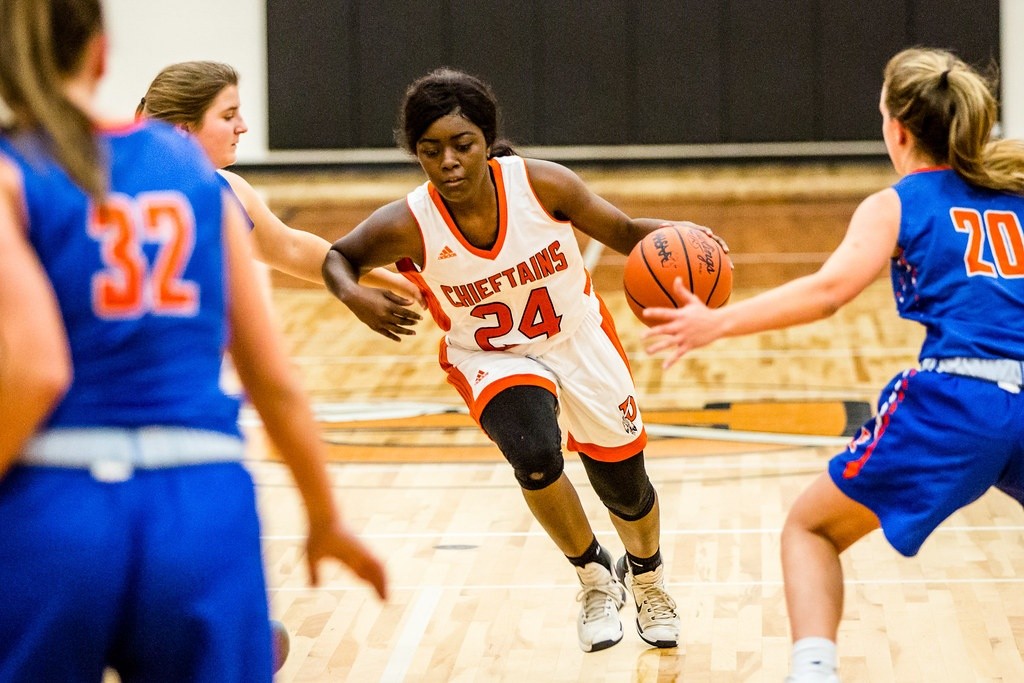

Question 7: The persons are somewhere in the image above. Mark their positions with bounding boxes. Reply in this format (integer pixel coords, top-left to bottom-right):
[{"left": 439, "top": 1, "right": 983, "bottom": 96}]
[
  {"left": 322, "top": 69, "right": 733, "bottom": 655},
  {"left": 0, "top": 0, "right": 388, "bottom": 683},
  {"left": 637, "top": 48, "right": 1024, "bottom": 681},
  {"left": 128, "top": 64, "right": 434, "bottom": 308}
]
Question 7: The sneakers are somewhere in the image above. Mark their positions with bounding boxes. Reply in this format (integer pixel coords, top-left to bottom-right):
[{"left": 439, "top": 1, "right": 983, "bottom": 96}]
[
  {"left": 575, "top": 562, "right": 626, "bottom": 654},
  {"left": 616, "top": 552, "right": 682, "bottom": 647}
]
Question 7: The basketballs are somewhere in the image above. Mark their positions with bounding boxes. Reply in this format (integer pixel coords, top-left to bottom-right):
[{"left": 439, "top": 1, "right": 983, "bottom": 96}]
[{"left": 620, "top": 224, "right": 733, "bottom": 336}]
